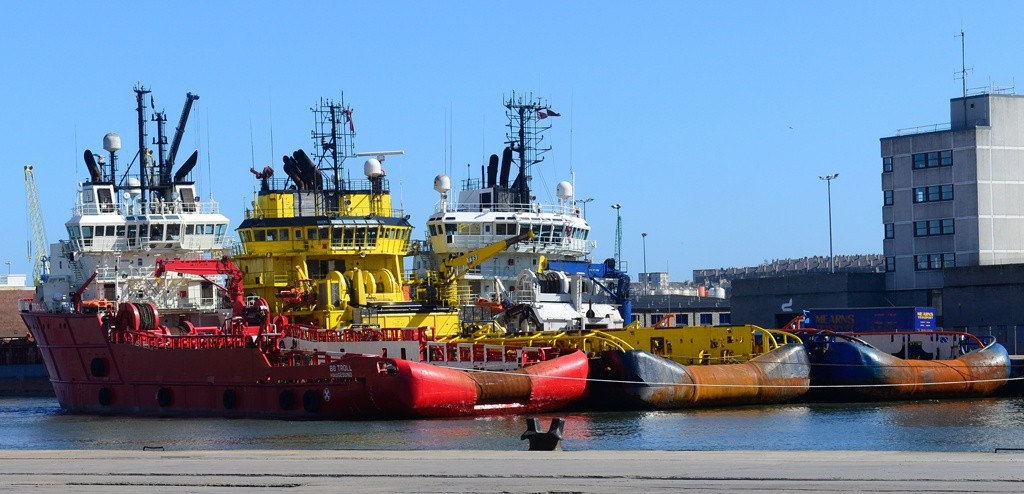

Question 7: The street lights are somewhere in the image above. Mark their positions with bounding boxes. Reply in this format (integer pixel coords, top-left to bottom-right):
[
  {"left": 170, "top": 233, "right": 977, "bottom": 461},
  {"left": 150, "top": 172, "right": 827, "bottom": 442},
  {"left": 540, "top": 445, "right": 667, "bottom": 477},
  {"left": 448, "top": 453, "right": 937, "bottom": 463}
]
[
  {"left": 818, "top": 172, "right": 842, "bottom": 275},
  {"left": 576, "top": 198, "right": 596, "bottom": 261},
  {"left": 642, "top": 233, "right": 648, "bottom": 295},
  {"left": 610, "top": 203, "right": 623, "bottom": 271}
]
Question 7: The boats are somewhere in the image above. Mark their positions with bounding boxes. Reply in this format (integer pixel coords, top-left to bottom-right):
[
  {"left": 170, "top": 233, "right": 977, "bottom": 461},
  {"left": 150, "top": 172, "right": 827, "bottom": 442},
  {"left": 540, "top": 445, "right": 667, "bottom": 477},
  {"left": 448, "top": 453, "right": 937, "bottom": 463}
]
[{"left": 17, "top": 76, "right": 1015, "bottom": 424}]
